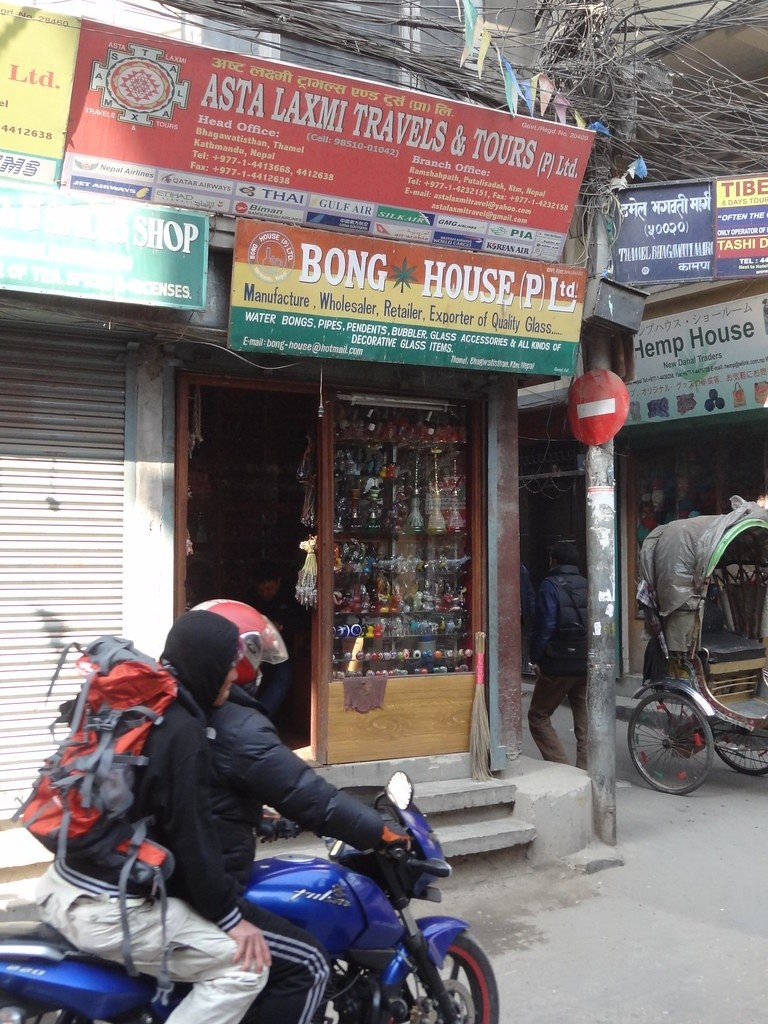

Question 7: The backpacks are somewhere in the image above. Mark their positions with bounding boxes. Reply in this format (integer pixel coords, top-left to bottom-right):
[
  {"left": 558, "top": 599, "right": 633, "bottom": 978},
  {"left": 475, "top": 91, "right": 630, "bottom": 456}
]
[{"left": 20, "top": 636, "right": 205, "bottom": 884}]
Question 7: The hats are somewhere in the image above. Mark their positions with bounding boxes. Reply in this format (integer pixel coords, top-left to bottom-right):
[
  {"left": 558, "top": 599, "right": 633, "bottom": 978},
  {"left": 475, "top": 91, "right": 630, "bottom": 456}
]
[{"left": 547, "top": 540, "right": 580, "bottom": 563}]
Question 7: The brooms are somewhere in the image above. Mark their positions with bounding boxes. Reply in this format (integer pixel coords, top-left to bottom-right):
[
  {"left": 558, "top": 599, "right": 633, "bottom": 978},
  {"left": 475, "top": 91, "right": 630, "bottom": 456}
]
[{"left": 470, "top": 632, "right": 494, "bottom": 782}]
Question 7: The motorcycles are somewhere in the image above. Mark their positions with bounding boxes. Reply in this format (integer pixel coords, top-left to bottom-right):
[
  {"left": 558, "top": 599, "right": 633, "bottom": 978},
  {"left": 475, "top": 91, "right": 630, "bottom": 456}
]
[{"left": 1, "top": 771, "right": 501, "bottom": 1023}]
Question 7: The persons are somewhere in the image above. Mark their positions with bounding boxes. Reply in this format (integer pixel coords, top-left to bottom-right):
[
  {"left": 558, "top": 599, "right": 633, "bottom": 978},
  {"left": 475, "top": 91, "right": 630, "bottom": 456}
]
[
  {"left": 39, "top": 598, "right": 413, "bottom": 1024},
  {"left": 518, "top": 539, "right": 588, "bottom": 771}
]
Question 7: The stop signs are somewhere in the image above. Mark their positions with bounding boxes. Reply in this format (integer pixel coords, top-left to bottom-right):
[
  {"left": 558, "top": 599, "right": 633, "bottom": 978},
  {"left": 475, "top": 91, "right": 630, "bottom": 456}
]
[{"left": 568, "top": 370, "right": 632, "bottom": 448}]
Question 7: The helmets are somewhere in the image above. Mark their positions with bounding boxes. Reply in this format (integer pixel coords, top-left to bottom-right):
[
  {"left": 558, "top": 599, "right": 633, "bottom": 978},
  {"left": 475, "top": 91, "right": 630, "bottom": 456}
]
[{"left": 189, "top": 599, "right": 290, "bottom": 697}]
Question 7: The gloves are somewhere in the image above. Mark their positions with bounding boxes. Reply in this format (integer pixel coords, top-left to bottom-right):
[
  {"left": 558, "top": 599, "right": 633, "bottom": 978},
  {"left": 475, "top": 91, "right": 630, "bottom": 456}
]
[
  {"left": 375, "top": 820, "right": 412, "bottom": 858},
  {"left": 262, "top": 804, "right": 283, "bottom": 840}
]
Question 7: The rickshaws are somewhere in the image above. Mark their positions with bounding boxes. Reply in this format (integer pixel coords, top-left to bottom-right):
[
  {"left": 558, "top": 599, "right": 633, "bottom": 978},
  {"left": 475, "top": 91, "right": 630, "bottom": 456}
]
[{"left": 624, "top": 502, "right": 767, "bottom": 795}]
